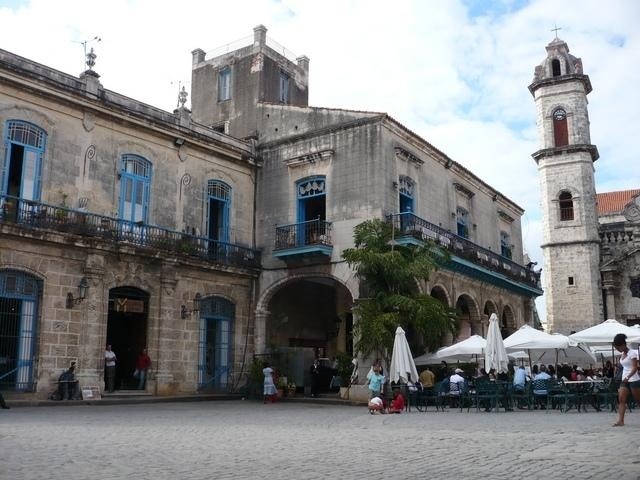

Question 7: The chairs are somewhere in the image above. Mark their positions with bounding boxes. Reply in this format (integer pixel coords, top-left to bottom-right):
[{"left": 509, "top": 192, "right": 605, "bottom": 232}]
[{"left": 382, "top": 371, "right": 640, "bottom": 417}]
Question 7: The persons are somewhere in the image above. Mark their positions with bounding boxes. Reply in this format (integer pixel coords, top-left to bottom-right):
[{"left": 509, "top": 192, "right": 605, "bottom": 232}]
[
  {"left": 262, "top": 362, "right": 279, "bottom": 404},
  {"left": 310, "top": 358, "right": 322, "bottom": 397},
  {"left": 365, "top": 333, "right": 640, "bottom": 426},
  {"left": 58, "top": 366, "right": 79, "bottom": 401},
  {"left": 133, "top": 348, "right": 151, "bottom": 390},
  {"left": 105, "top": 344, "right": 118, "bottom": 393}
]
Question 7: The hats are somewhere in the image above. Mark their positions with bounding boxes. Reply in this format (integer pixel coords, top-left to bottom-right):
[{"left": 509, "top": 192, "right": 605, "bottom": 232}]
[{"left": 455, "top": 368, "right": 464, "bottom": 374}]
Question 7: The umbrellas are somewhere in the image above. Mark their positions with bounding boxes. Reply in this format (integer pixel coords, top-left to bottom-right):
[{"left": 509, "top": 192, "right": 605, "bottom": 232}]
[{"left": 389, "top": 313, "right": 640, "bottom": 411}]
